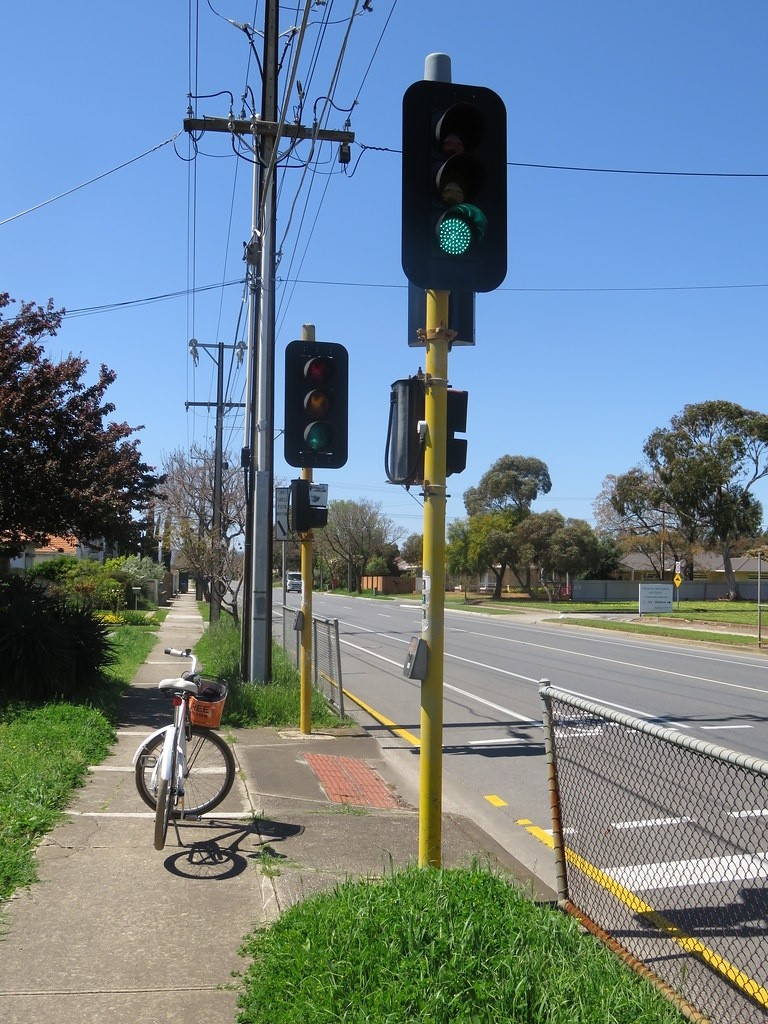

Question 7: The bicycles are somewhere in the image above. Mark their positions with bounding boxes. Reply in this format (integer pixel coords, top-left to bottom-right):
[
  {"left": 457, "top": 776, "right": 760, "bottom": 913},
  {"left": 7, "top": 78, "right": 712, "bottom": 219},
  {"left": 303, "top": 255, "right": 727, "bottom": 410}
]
[{"left": 131, "top": 646, "right": 237, "bottom": 849}]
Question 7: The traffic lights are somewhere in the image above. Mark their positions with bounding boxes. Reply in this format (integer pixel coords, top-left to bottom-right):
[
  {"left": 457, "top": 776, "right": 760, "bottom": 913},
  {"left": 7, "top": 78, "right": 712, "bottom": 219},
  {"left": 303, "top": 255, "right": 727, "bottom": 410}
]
[
  {"left": 284, "top": 340, "right": 348, "bottom": 469},
  {"left": 384, "top": 376, "right": 467, "bottom": 484},
  {"left": 289, "top": 479, "right": 332, "bottom": 535},
  {"left": 401, "top": 79, "right": 509, "bottom": 291}
]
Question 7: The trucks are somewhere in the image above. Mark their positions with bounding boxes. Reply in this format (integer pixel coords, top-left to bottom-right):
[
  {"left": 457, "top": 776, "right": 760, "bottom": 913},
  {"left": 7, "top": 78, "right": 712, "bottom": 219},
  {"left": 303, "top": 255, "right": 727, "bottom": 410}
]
[{"left": 287, "top": 571, "right": 301, "bottom": 594}]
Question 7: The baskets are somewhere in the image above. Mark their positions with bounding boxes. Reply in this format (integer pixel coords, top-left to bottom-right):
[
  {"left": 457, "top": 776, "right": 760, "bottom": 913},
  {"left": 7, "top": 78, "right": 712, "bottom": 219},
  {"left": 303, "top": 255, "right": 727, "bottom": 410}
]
[{"left": 189, "top": 679, "right": 227, "bottom": 728}]
